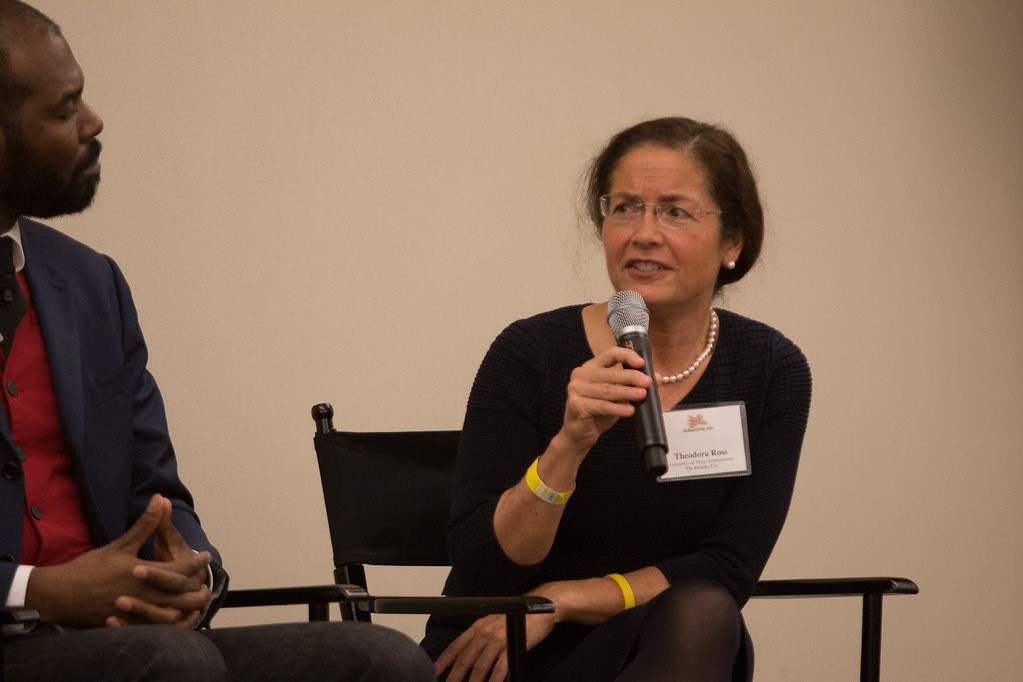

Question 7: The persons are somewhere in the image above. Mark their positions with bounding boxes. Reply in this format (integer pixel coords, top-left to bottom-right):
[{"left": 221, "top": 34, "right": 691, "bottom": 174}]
[
  {"left": 420, "top": 117, "right": 813, "bottom": 682},
  {"left": 0, "top": 0, "right": 448, "bottom": 682}
]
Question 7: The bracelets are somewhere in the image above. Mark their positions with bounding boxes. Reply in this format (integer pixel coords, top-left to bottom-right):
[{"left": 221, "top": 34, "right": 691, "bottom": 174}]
[
  {"left": 525, "top": 455, "right": 577, "bottom": 505},
  {"left": 604, "top": 572, "right": 636, "bottom": 611}
]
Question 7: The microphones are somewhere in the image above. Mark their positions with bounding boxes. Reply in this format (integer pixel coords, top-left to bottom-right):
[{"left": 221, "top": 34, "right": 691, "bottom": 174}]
[{"left": 607, "top": 289, "right": 671, "bottom": 477}]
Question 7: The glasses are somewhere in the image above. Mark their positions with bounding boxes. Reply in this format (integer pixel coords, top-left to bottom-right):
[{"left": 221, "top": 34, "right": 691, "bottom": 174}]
[{"left": 599, "top": 193, "right": 725, "bottom": 229}]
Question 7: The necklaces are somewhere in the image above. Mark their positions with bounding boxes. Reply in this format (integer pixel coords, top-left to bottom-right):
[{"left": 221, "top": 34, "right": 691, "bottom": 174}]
[{"left": 661, "top": 304, "right": 718, "bottom": 384}]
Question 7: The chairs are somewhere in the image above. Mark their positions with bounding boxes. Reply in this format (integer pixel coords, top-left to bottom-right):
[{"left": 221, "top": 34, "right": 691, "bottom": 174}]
[{"left": 309, "top": 399, "right": 920, "bottom": 682}]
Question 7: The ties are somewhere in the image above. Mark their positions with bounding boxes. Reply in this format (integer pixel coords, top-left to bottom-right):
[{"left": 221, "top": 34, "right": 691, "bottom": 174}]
[{"left": 0, "top": 236, "right": 26, "bottom": 360}]
